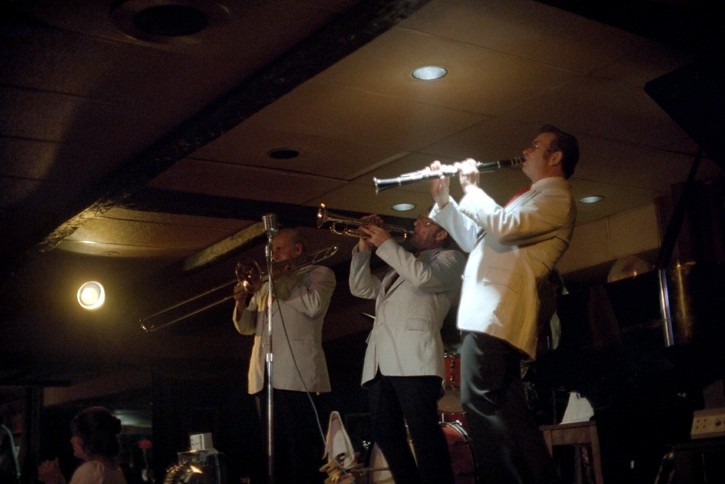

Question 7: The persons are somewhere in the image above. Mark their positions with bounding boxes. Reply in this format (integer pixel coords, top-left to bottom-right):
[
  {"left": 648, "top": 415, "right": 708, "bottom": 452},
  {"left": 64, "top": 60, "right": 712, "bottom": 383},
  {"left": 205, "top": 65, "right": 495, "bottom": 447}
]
[
  {"left": 38, "top": 407, "right": 127, "bottom": 484},
  {"left": 349, "top": 203, "right": 459, "bottom": 484},
  {"left": 233, "top": 228, "right": 336, "bottom": 484},
  {"left": 426, "top": 125, "right": 579, "bottom": 484}
]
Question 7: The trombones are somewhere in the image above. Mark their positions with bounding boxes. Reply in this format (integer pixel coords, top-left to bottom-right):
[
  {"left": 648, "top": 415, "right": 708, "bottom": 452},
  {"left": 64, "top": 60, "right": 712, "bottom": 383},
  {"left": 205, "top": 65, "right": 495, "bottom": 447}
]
[{"left": 135, "top": 247, "right": 338, "bottom": 334}]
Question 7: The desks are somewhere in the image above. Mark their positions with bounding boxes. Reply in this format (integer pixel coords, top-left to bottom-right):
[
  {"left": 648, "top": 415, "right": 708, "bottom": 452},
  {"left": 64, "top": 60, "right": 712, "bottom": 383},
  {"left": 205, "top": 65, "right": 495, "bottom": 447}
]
[{"left": 539, "top": 421, "right": 603, "bottom": 484}]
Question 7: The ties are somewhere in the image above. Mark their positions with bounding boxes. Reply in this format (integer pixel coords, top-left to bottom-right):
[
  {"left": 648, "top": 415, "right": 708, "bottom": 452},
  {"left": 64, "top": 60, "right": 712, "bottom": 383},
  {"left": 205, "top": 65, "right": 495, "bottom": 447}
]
[{"left": 506, "top": 187, "right": 531, "bottom": 207}]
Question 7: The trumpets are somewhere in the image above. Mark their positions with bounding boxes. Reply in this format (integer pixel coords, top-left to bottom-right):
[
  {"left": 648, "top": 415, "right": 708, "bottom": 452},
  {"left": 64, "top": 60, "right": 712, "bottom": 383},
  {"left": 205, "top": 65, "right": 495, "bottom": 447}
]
[
  {"left": 373, "top": 156, "right": 523, "bottom": 193},
  {"left": 315, "top": 203, "right": 416, "bottom": 241}
]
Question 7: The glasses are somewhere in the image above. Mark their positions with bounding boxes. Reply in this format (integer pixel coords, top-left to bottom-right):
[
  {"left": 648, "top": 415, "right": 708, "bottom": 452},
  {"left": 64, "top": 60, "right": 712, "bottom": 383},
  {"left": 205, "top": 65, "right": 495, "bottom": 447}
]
[{"left": 418, "top": 214, "right": 444, "bottom": 230}]
